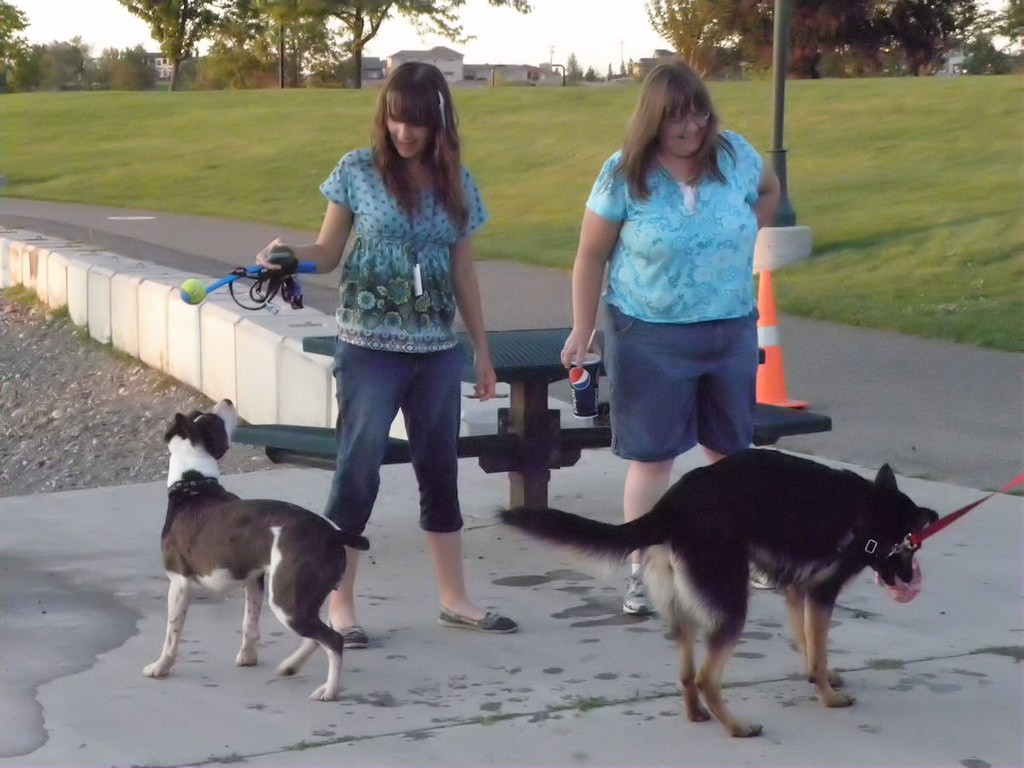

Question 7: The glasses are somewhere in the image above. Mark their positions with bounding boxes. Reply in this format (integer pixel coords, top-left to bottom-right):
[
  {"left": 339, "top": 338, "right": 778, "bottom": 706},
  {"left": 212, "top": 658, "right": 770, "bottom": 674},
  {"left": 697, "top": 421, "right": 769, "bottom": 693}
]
[{"left": 666, "top": 109, "right": 710, "bottom": 128}]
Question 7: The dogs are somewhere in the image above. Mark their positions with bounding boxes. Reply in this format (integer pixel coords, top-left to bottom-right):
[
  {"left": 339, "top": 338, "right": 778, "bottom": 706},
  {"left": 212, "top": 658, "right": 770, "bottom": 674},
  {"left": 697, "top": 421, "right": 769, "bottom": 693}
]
[
  {"left": 486, "top": 445, "right": 941, "bottom": 740},
  {"left": 139, "top": 395, "right": 373, "bottom": 705}
]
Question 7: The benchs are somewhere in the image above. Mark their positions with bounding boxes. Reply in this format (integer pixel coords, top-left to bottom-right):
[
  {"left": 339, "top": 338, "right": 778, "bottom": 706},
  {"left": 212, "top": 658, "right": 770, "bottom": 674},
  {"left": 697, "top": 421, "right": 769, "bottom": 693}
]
[
  {"left": 231, "top": 424, "right": 410, "bottom": 462},
  {"left": 752, "top": 402, "right": 832, "bottom": 446}
]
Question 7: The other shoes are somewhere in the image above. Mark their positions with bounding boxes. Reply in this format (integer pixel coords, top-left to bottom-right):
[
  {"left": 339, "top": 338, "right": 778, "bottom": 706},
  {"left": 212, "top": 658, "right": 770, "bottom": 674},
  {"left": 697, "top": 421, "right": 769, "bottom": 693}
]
[
  {"left": 326, "top": 618, "right": 368, "bottom": 648},
  {"left": 437, "top": 606, "right": 518, "bottom": 633}
]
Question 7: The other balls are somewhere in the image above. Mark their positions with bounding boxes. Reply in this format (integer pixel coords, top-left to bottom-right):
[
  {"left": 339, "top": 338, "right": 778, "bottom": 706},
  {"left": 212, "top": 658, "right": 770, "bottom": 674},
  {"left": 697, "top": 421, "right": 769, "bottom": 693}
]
[{"left": 178, "top": 278, "right": 207, "bottom": 306}]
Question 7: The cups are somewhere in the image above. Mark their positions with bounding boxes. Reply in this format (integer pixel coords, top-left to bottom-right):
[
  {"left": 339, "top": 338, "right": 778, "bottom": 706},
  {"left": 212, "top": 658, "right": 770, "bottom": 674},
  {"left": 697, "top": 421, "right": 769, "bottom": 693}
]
[{"left": 568, "top": 353, "right": 601, "bottom": 419}]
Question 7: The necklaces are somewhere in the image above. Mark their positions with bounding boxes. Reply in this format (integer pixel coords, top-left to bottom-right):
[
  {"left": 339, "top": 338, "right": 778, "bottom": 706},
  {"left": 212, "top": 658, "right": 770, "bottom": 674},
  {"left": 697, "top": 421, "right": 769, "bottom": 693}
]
[{"left": 406, "top": 181, "right": 438, "bottom": 297}]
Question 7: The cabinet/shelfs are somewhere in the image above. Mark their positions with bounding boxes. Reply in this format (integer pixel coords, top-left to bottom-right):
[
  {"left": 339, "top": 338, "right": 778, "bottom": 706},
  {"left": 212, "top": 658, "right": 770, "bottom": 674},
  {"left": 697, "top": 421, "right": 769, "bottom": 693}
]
[{"left": 302, "top": 326, "right": 606, "bottom": 511}]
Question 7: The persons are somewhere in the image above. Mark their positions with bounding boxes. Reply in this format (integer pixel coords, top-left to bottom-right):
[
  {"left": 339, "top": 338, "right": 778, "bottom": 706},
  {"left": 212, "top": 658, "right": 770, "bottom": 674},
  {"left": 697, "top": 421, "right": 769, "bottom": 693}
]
[
  {"left": 254, "top": 61, "right": 520, "bottom": 649},
  {"left": 560, "top": 59, "right": 782, "bottom": 614}
]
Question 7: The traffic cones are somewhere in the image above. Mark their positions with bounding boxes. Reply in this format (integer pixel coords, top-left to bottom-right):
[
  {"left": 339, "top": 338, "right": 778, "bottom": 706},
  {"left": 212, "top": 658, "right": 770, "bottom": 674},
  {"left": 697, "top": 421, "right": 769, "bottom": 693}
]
[{"left": 753, "top": 268, "right": 810, "bottom": 408}]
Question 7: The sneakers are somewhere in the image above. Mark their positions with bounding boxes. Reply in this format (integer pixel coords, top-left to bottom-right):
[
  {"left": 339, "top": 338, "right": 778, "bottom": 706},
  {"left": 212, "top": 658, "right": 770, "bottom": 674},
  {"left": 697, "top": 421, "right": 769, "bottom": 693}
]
[
  {"left": 622, "top": 574, "right": 656, "bottom": 614},
  {"left": 748, "top": 561, "right": 777, "bottom": 589}
]
